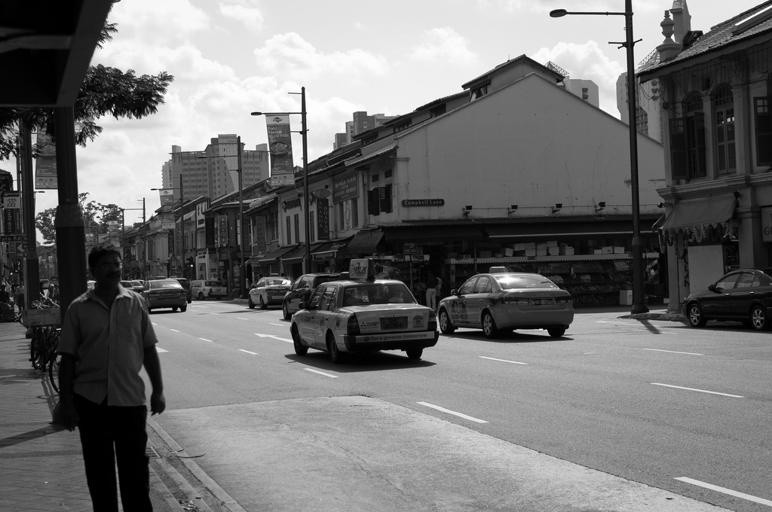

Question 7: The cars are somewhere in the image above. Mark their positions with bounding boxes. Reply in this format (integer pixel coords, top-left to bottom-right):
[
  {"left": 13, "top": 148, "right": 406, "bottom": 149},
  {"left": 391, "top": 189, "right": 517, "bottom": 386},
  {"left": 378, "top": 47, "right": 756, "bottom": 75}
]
[
  {"left": 190, "top": 279, "right": 228, "bottom": 301},
  {"left": 681, "top": 266, "right": 772, "bottom": 331},
  {"left": 289, "top": 258, "right": 439, "bottom": 365},
  {"left": 437, "top": 271, "right": 575, "bottom": 339},
  {"left": 282, "top": 271, "right": 350, "bottom": 321},
  {"left": 248, "top": 275, "right": 292, "bottom": 310},
  {"left": 142, "top": 279, "right": 187, "bottom": 313}
]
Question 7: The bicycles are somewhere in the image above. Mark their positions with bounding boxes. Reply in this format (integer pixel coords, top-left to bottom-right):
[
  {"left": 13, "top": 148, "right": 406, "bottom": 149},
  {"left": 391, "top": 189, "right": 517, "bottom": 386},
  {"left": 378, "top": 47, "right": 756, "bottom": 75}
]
[{"left": 23, "top": 304, "right": 63, "bottom": 393}]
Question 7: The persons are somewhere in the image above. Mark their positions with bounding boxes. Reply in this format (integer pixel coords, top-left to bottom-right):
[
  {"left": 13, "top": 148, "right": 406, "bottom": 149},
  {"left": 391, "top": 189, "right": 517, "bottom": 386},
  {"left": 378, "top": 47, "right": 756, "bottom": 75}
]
[
  {"left": 57, "top": 243, "right": 165, "bottom": 512},
  {"left": 0, "top": 277, "right": 25, "bottom": 316},
  {"left": 435, "top": 275, "right": 442, "bottom": 308},
  {"left": 426, "top": 271, "right": 439, "bottom": 310}
]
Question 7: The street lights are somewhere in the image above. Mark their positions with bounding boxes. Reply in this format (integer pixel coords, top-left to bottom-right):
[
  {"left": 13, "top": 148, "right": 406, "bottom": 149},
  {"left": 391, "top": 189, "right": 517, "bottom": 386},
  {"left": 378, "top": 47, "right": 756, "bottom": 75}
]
[
  {"left": 250, "top": 86, "right": 312, "bottom": 275},
  {"left": 150, "top": 173, "right": 185, "bottom": 279},
  {"left": 196, "top": 136, "right": 249, "bottom": 299},
  {"left": 549, "top": 0, "right": 651, "bottom": 315}
]
